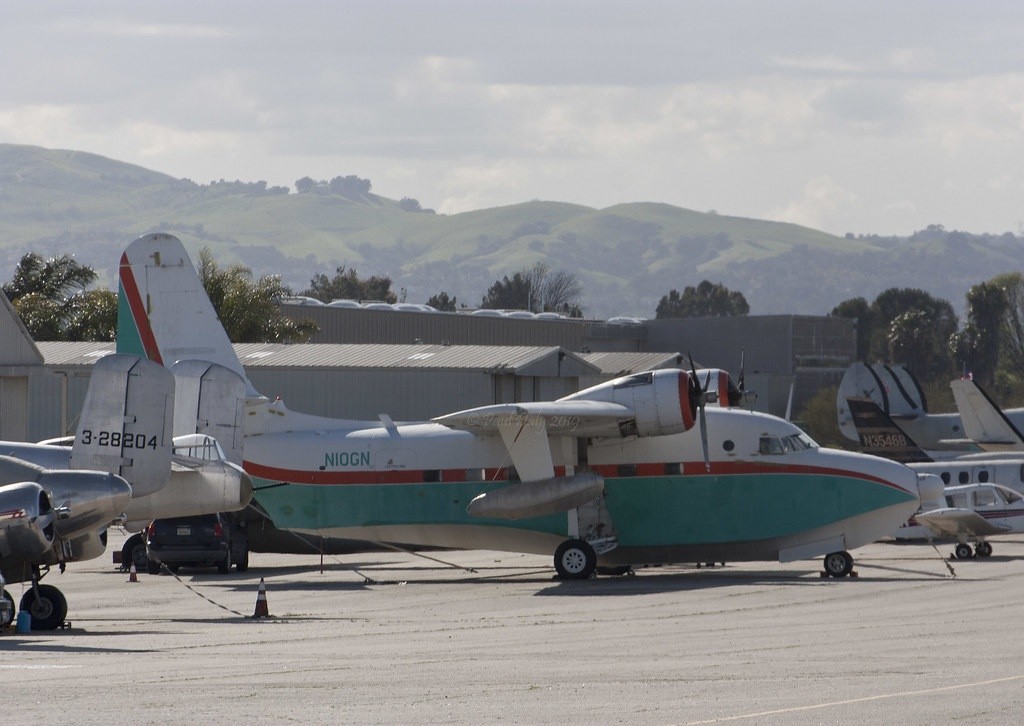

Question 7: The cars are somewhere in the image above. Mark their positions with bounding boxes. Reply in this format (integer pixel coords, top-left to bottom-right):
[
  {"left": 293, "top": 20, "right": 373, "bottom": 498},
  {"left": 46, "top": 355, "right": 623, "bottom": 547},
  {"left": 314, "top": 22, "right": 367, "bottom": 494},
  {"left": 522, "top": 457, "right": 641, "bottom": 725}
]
[{"left": 146, "top": 514, "right": 249, "bottom": 576}]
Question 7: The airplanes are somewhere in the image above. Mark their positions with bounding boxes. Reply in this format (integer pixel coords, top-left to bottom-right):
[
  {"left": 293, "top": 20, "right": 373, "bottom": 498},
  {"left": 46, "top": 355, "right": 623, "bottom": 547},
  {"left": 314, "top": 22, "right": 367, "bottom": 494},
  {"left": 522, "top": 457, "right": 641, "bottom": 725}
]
[
  {"left": 0, "top": 356, "right": 292, "bottom": 635},
  {"left": 836, "top": 362, "right": 1024, "bottom": 561},
  {"left": 113, "top": 233, "right": 920, "bottom": 582}
]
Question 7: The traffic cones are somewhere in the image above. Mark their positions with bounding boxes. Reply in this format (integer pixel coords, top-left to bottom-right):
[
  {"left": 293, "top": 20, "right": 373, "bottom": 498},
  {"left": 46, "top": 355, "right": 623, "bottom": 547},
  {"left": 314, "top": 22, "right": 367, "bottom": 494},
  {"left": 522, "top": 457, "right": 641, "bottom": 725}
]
[
  {"left": 127, "top": 557, "right": 141, "bottom": 584},
  {"left": 247, "top": 576, "right": 273, "bottom": 619}
]
